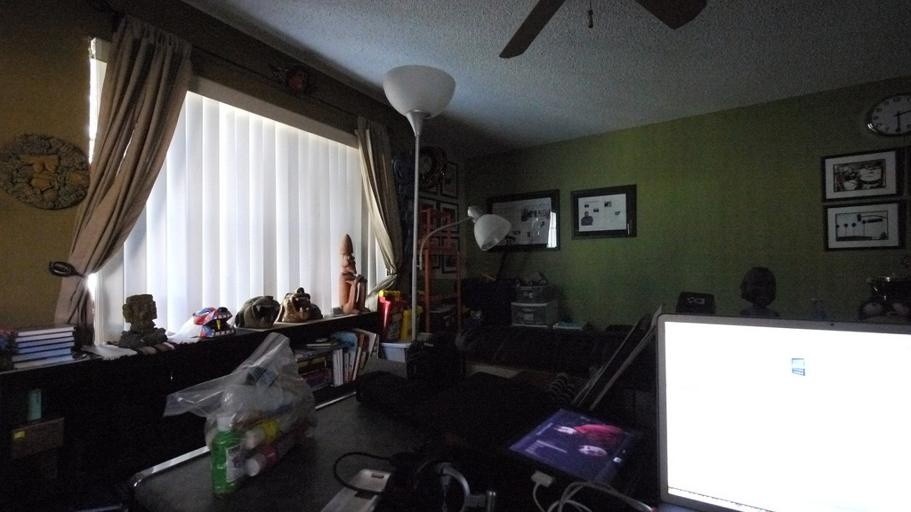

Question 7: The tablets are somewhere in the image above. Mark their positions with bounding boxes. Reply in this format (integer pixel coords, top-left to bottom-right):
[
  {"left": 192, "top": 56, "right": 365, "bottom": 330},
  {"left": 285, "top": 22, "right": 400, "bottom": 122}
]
[{"left": 508, "top": 408, "right": 654, "bottom": 487}]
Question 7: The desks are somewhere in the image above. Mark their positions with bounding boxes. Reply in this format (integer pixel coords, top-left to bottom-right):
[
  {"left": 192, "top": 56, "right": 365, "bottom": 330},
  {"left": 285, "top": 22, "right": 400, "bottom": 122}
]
[{"left": 128, "top": 385, "right": 637, "bottom": 510}]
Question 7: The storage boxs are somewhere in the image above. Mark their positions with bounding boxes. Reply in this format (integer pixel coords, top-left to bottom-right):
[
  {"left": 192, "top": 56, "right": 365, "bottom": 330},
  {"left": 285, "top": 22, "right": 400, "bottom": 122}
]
[
  {"left": 382, "top": 343, "right": 413, "bottom": 363},
  {"left": 291, "top": 351, "right": 334, "bottom": 394},
  {"left": 510, "top": 282, "right": 589, "bottom": 330},
  {"left": 6, "top": 415, "right": 67, "bottom": 458}
]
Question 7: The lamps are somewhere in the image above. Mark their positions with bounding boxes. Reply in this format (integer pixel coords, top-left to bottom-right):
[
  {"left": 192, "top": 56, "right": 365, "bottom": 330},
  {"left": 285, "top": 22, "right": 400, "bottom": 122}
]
[
  {"left": 423, "top": 205, "right": 512, "bottom": 251},
  {"left": 382, "top": 66, "right": 456, "bottom": 339}
]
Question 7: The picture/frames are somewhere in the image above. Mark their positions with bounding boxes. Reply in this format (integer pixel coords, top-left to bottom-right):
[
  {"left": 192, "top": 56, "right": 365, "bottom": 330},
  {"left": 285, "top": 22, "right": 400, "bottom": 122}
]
[
  {"left": 419, "top": 237, "right": 463, "bottom": 272},
  {"left": 421, "top": 198, "right": 462, "bottom": 235},
  {"left": 488, "top": 190, "right": 560, "bottom": 251},
  {"left": 819, "top": 147, "right": 905, "bottom": 203},
  {"left": 570, "top": 184, "right": 637, "bottom": 238},
  {"left": 822, "top": 202, "right": 906, "bottom": 252},
  {"left": 420, "top": 159, "right": 460, "bottom": 198}
]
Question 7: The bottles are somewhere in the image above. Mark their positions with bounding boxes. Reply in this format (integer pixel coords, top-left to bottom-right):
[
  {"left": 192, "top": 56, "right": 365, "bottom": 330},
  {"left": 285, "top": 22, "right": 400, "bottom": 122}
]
[{"left": 210, "top": 413, "right": 244, "bottom": 495}]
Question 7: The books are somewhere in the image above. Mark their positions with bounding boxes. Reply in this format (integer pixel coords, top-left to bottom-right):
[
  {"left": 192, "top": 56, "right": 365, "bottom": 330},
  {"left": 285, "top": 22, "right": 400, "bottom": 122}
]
[
  {"left": 0, "top": 322, "right": 78, "bottom": 370},
  {"left": 291, "top": 326, "right": 380, "bottom": 391}
]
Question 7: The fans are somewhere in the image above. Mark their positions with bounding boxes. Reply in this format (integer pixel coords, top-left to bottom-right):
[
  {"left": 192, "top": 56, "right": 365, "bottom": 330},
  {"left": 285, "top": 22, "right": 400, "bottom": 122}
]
[{"left": 498, "top": 2, "right": 711, "bottom": 60}]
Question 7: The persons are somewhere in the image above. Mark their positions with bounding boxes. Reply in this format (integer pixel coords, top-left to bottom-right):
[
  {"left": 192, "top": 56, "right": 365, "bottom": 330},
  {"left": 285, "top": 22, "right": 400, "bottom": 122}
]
[{"left": 581, "top": 212, "right": 593, "bottom": 226}]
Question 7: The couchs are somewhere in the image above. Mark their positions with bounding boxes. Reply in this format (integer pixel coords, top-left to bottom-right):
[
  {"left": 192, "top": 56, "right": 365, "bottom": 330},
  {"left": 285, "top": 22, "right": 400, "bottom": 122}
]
[{"left": 406, "top": 318, "right": 657, "bottom": 414}]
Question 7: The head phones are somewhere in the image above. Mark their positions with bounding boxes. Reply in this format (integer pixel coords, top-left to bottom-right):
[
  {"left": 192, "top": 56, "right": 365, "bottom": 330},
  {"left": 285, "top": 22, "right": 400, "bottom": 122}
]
[{"left": 332, "top": 452, "right": 420, "bottom": 505}]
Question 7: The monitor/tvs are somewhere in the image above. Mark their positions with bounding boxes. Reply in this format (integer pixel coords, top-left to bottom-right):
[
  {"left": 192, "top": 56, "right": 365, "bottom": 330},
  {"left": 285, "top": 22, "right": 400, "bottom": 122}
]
[{"left": 657, "top": 313, "right": 910, "bottom": 512}]
